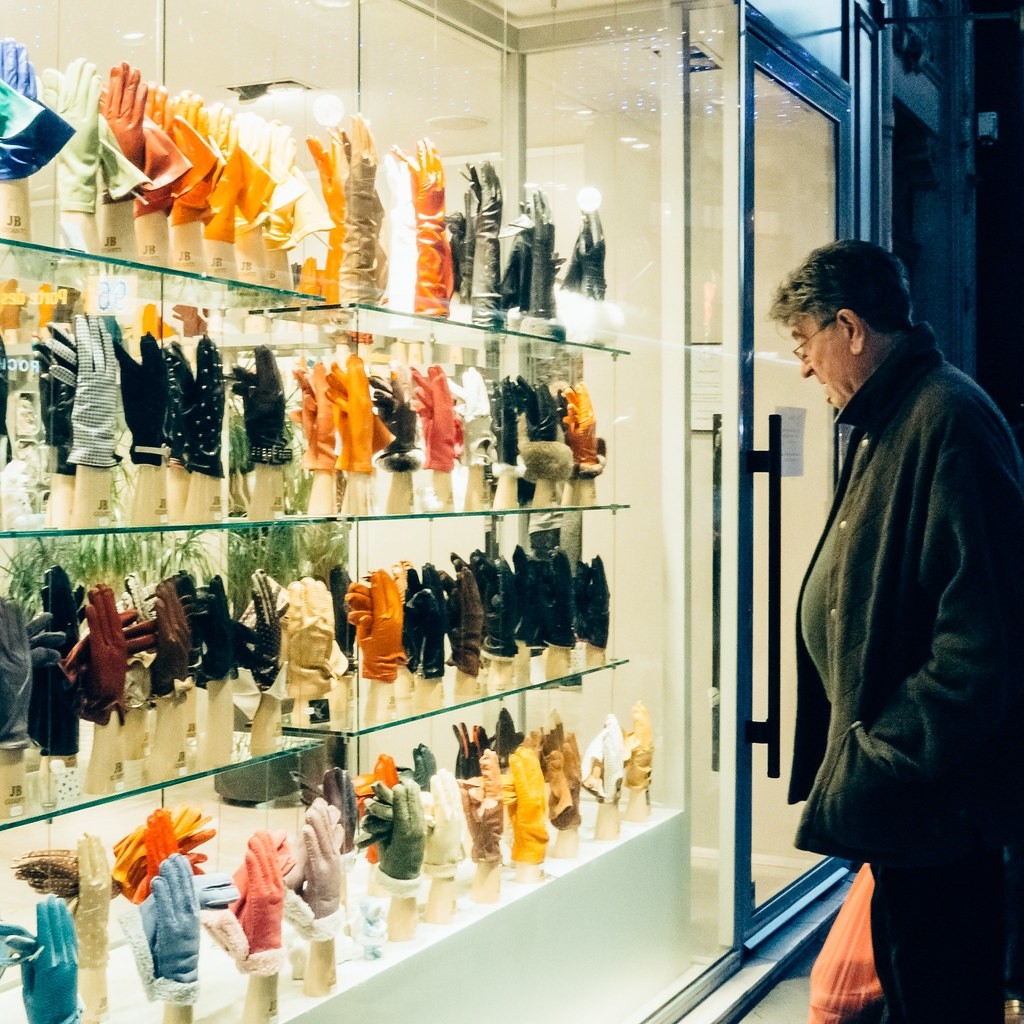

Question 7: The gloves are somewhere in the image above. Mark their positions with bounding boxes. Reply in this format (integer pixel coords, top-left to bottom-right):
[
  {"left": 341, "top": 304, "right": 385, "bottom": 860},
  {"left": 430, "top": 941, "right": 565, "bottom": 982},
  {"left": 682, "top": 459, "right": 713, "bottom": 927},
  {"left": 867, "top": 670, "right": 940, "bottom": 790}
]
[{"left": 0, "top": 36, "right": 654, "bottom": 1024}]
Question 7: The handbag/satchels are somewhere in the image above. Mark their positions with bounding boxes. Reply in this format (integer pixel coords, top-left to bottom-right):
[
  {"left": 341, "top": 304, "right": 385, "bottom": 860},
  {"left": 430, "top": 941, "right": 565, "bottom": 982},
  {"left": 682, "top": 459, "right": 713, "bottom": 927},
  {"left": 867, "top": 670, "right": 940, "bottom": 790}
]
[{"left": 808, "top": 863, "right": 884, "bottom": 1024}]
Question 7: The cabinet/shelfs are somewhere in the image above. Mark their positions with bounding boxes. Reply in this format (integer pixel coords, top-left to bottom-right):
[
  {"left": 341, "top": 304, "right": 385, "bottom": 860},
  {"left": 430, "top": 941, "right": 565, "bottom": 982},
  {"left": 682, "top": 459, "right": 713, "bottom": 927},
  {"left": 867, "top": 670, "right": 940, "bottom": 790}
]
[{"left": 0, "top": 237, "right": 633, "bottom": 834}]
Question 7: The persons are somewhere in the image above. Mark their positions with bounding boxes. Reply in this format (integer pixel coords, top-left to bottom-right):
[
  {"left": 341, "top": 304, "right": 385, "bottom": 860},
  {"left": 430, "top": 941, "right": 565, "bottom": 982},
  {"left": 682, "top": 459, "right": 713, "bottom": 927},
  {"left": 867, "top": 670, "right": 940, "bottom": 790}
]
[{"left": 769, "top": 241, "right": 1024, "bottom": 1024}]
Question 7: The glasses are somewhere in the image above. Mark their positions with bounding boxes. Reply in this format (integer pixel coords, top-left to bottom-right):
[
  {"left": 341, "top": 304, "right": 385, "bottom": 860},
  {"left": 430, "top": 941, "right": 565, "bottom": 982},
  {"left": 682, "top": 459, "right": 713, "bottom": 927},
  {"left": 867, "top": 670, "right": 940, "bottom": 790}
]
[{"left": 794, "top": 316, "right": 836, "bottom": 363}]
